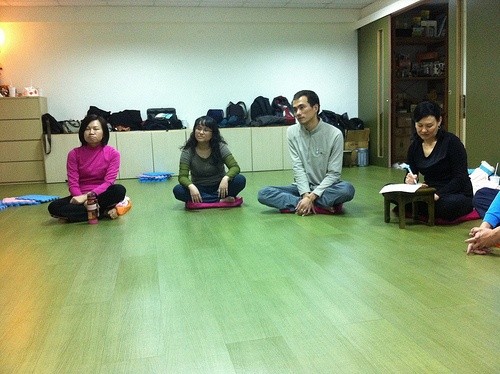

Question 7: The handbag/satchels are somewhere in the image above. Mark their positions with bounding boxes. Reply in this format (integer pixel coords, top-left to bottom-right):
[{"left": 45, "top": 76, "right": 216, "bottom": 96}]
[
  {"left": 41, "top": 112, "right": 63, "bottom": 135},
  {"left": 349, "top": 118, "right": 366, "bottom": 130},
  {"left": 61, "top": 120, "right": 82, "bottom": 134},
  {"left": 320, "top": 110, "right": 348, "bottom": 141},
  {"left": 86, "top": 106, "right": 185, "bottom": 131}
]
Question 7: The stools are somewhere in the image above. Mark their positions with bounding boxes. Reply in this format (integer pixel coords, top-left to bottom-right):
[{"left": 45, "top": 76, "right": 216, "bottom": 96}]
[{"left": 384, "top": 186, "right": 437, "bottom": 228}]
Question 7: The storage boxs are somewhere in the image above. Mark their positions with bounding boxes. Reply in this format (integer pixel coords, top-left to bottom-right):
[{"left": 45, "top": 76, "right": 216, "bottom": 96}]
[
  {"left": 392, "top": 115, "right": 416, "bottom": 163},
  {"left": 343, "top": 128, "right": 371, "bottom": 167}
]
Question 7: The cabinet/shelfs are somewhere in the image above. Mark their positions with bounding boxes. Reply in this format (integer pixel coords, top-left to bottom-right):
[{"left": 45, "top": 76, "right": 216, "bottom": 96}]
[
  {"left": 0, "top": 95, "right": 47, "bottom": 186},
  {"left": 392, "top": 3, "right": 448, "bottom": 166}
]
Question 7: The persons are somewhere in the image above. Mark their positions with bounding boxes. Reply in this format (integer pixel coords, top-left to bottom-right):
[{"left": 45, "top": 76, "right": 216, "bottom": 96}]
[
  {"left": 256, "top": 88, "right": 355, "bottom": 215},
  {"left": 381, "top": 100, "right": 475, "bottom": 226},
  {"left": 48, "top": 113, "right": 132, "bottom": 223},
  {"left": 463, "top": 187, "right": 500, "bottom": 257},
  {"left": 172, "top": 115, "right": 246, "bottom": 210}
]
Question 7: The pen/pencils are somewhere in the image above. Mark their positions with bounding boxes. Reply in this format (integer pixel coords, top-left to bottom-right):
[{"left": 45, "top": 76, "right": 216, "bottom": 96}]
[{"left": 405, "top": 164, "right": 419, "bottom": 184}]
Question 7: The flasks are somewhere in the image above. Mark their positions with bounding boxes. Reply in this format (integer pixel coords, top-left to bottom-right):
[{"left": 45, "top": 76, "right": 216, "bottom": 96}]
[{"left": 86, "top": 194, "right": 100, "bottom": 224}]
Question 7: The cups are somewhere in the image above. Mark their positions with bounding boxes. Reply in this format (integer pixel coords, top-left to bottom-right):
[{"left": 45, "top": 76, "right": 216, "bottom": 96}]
[
  {"left": 489, "top": 175, "right": 500, "bottom": 185},
  {"left": 8, "top": 87, "right": 15, "bottom": 98}
]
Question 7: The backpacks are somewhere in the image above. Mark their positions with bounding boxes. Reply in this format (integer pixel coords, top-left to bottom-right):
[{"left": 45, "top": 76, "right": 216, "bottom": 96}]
[
  {"left": 250, "top": 96, "right": 294, "bottom": 123},
  {"left": 226, "top": 101, "right": 248, "bottom": 127},
  {"left": 207, "top": 108, "right": 225, "bottom": 128}
]
